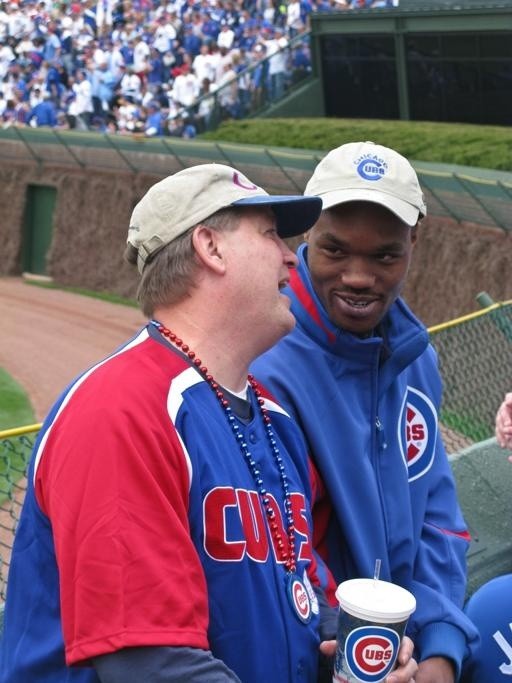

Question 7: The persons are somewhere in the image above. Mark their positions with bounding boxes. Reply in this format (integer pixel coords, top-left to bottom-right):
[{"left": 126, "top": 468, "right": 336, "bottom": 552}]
[
  {"left": 463, "top": 393, "right": 512, "bottom": 682},
  {"left": 0, "top": 0, "right": 399, "bottom": 142},
  {"left": 247, "top": 141, "right": 482, "bottom": 682},
  {"left": 1, "top": 162, "right": 419, "bottom": 682}
]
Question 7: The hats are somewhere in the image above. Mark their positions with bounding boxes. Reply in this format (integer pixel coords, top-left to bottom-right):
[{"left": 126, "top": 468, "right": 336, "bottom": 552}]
[
  {"left": 303, "top": 137, "right": 430, "bottom": 229},
  {"left": 120, "top": 163, "right": 325, "bottom": 273}
]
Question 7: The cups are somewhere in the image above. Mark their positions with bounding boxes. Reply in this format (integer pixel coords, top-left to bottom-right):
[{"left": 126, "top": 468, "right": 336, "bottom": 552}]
[{"left": 328, "top": 578, "right": 418, "bottom": 683}]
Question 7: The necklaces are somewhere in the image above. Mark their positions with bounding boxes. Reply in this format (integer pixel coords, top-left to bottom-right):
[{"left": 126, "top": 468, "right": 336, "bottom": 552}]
[{"left": 150, "top": 322, "right": 312, "bottom": 625}]
